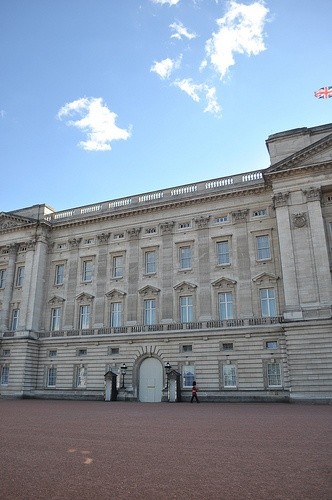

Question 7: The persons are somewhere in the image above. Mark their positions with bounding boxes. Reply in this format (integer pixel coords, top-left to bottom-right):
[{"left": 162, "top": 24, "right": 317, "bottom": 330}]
[{"left": 190, "top": 381, "right": 200, "bottom": 403}]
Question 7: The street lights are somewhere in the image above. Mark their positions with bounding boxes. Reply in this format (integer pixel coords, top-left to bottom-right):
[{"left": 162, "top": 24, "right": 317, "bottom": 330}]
[
  {"left": 163, "top": 362, "right": 172, "bottom": 391},
  {"left": 121, "top": 363, "right": 128, "bottom": 387}
]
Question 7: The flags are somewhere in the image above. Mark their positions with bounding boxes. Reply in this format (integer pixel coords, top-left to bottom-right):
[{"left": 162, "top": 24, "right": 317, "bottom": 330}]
[{"left": 313, "top": 86, "right": 332, "bottom": 99}]
